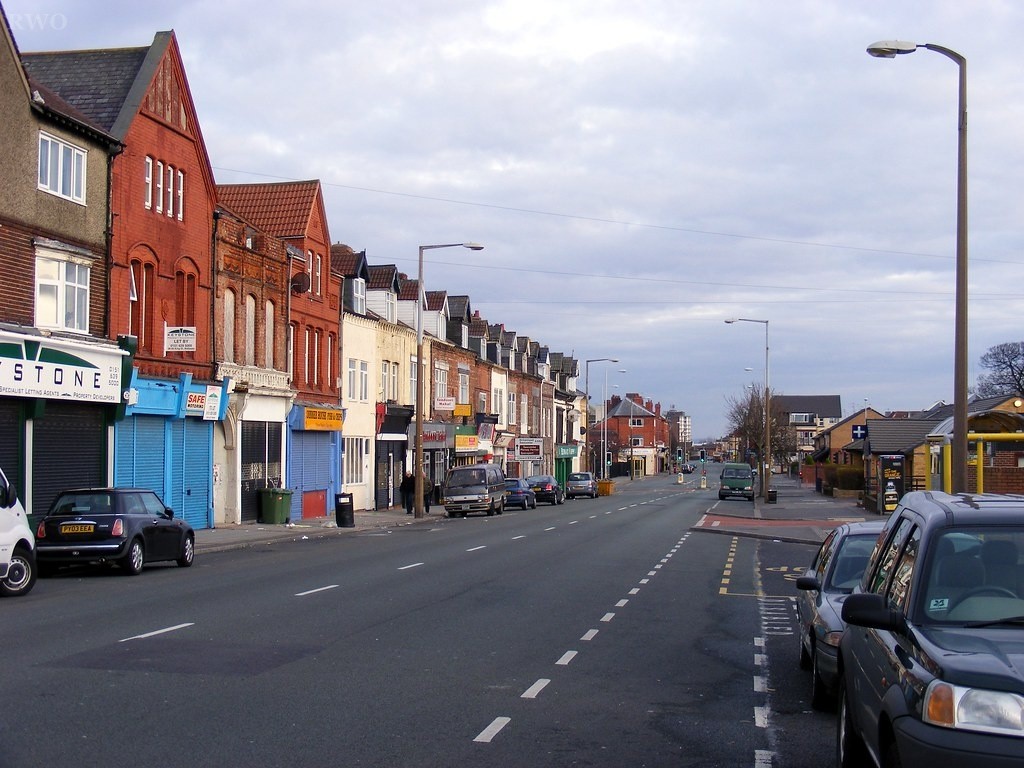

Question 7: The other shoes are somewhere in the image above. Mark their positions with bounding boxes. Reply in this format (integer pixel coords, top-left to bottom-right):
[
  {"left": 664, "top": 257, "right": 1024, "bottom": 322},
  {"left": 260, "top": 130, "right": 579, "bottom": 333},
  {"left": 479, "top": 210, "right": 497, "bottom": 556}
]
[{"left": 406, "top": 511, "right": 412, "bottom": 514}]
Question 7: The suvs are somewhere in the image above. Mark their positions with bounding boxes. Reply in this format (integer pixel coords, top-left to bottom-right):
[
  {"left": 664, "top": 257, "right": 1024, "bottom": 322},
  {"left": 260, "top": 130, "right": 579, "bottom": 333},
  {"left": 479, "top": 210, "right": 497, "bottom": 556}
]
[{"left": 833, "top": 488, "right": 1024, "bottom": 768}]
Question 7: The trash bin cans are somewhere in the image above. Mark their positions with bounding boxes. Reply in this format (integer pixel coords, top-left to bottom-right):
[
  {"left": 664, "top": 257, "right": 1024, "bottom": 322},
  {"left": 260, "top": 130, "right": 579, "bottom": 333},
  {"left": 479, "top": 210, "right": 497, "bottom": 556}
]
[
  {"left": 815, "top": 478, "right": 822, "bottom": 492},
  {"left": 260, "top": 488, "right": 294, "bottom": 524},
  {"left": 768, "top": 491, "right": 777, "bottom": 504},
  {"left": 335, "top": 492, "right": 355, "bottom": 528},
  {"left": 435, "top": 486, "right": 444, "bottom": 505}
]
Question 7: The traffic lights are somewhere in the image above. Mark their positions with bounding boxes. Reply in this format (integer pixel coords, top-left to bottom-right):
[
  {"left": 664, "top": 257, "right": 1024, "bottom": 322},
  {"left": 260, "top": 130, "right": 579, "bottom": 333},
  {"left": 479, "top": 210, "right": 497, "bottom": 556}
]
[
  {"left": 700, "top": 450, "right": 706, "bottom": 463},
  {"left": 677, "top": 449, "right": 683, "bottom": 463},
  {"left": 606, "top": 452, "right": 612, "bottom": 466}
]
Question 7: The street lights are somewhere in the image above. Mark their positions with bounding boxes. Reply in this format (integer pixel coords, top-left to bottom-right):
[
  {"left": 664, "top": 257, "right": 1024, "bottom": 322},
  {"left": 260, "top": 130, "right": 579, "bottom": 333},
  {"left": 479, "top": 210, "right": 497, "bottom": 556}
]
[
  {"left": 585, "top": 358, "right": 620, "bottom": 472},
  {"left": 866, "top": 37, "right": 971, "bottom": 491},
  {"left": 414, "top": 243, "right": 486, "bottom": 519},
  {"left": 723, "top": 317, "right": 772, "bottom": 504},
  {"left": 604, "top": 366, "right": 627, "bottom": 479},
  {"left": 744, "top": 367, "right": 771, "bottom": 498},
  {"left": 600, "top": 383, "right": 618, "bottom": 481}
]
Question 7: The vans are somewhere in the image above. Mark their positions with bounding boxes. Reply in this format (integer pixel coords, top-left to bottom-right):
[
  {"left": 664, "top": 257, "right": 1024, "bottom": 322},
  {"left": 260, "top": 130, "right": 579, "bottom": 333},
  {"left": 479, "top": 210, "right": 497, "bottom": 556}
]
[
  {"left": 718, "top": 462, "right": 757, "bottom": 502},
  {"left": 0, "top": 466, "right": 38, "bottom": 599},
  {"left": 438, "top": 462, "right": 508, "bottom": 519}
]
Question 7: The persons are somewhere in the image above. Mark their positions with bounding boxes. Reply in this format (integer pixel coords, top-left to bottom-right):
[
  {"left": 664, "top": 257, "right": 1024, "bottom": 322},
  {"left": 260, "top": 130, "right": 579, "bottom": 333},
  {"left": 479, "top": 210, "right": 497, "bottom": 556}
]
[
  {"left": 399, "top": 472, "right": 415, "bottom": 514},
  {"left": 502, "top": 470, "right": 506, "bottom": 478},
  {"left": 422, "top": 472, "right": 432, "bottom": 513}
]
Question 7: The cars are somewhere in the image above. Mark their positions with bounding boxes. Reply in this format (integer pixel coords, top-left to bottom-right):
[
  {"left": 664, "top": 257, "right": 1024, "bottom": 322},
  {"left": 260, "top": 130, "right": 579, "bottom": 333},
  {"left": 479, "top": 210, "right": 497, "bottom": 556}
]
[
  {"left": 795, "top": 518, "right": 985, "bottom": 715},
  {"left": 526, "top": 474, "right": 565, "bottom": 505},
  {"left": 34, "top": 487, "right": 196, "bottom": 576},
  {"left": 504, "top": 477, "right": 537, "bottom": 510},
  {"left": 566, "top": 471, "right": 599, "bottom": 500}
]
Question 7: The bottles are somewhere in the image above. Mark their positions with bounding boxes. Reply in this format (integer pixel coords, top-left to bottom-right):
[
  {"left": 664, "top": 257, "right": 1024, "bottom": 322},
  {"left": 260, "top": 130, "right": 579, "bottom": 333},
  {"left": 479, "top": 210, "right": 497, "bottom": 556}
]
[{"left": 885, "top": 482, "right": 898, "bottom": 511}]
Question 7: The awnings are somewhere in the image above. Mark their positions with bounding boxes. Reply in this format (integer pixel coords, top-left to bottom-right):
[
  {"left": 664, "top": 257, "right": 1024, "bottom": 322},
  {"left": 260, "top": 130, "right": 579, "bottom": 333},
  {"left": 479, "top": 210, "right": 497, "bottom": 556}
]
[{"left": 802, "top": 447, "right": 829, "bottom": 461}]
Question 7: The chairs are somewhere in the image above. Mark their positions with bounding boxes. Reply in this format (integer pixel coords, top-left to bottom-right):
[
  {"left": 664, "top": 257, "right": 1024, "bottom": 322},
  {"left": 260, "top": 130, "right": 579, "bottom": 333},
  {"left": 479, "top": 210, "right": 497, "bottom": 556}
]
[
  {"left": 934, "top": 554, "right": 986, "bottom": 607},
  {"left": 981, "top": 541, "right": 1018, "bottom": 594}
]
